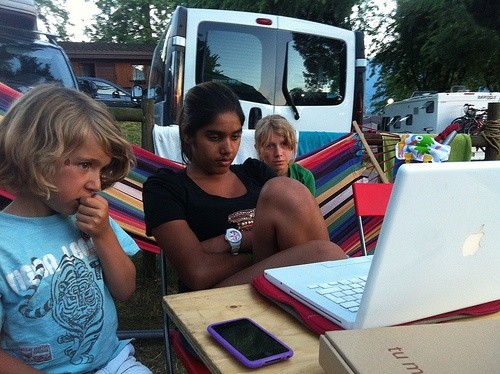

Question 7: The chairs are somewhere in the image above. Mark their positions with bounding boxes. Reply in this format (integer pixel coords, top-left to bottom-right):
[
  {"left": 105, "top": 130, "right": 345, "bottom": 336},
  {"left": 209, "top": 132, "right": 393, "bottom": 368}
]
[
  {"left": 160, "top": 245, "right": 212, "bottom": 374},
  {"left": 352, "top": 183, "right": 396, "bottom": 256}
]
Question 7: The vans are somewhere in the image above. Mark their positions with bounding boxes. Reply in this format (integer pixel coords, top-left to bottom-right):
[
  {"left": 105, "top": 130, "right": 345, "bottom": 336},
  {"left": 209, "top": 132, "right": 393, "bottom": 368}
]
[{"left": 132, "top": 6, "right": 356, "bottom": 166}]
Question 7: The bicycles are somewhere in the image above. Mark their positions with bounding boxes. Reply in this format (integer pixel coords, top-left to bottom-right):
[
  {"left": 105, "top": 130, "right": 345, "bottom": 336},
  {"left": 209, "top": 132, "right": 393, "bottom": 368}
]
[{"left": 452, "top": 103, "right": 488, "bottom": 137}]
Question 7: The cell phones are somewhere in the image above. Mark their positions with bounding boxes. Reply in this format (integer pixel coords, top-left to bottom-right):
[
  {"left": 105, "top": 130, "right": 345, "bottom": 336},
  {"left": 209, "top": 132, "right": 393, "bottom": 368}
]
[{"left": 207, "top": 317, "right": 293, "bottom": 369}]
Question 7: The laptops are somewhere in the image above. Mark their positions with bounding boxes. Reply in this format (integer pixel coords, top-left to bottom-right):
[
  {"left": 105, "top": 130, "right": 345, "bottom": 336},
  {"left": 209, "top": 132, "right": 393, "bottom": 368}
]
[{"left": 262, "top": 160, "right": 500, "bottom": 331}]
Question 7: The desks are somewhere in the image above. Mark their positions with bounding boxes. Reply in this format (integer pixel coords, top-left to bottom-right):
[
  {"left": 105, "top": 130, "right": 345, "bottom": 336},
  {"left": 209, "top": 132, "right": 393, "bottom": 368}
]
[{"left": 162, "top": 283, "right": 328, "bottom": 374}]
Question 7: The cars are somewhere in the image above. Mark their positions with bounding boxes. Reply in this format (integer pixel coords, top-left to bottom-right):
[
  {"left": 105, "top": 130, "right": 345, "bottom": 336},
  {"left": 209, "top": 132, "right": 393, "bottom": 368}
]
[{"left": 76, "top": 77, "right": 141, "bottom": 108}]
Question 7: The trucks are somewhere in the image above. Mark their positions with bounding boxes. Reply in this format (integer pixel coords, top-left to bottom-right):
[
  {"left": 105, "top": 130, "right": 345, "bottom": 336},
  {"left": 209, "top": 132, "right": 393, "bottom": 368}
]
[{"left": 377, "top": 85, "right": 500, "bottom": 161}]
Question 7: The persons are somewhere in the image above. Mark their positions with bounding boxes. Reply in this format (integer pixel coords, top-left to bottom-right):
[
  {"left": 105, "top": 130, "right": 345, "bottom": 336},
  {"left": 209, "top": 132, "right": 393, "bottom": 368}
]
[
  {"left": 254, "top": 114, "right": 316, "bottom": 196},
  {"left": 0, "top": 87, "right": 154, "bottom": 373},
  {"left": 142, "top": 81, "right": 348, "bottom": 294}
]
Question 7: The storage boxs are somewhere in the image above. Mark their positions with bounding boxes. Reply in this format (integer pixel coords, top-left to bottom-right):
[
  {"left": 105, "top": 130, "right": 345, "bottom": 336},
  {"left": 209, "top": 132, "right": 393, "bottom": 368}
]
[{"left": 319, "top": 311, "right": 500, "bottom": 374}]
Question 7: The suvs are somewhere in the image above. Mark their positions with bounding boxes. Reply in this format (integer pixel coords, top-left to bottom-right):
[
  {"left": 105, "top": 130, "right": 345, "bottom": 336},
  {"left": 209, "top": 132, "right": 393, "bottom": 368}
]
[{"left": 0, "top": 26, "right": 80, "bottom": 92}]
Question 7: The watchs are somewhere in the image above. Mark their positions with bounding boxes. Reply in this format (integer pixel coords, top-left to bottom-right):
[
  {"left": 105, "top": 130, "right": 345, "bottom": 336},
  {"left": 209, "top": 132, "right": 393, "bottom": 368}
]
[{"left": 224, "top": 228, "right": 243, "bottom": 257}]
